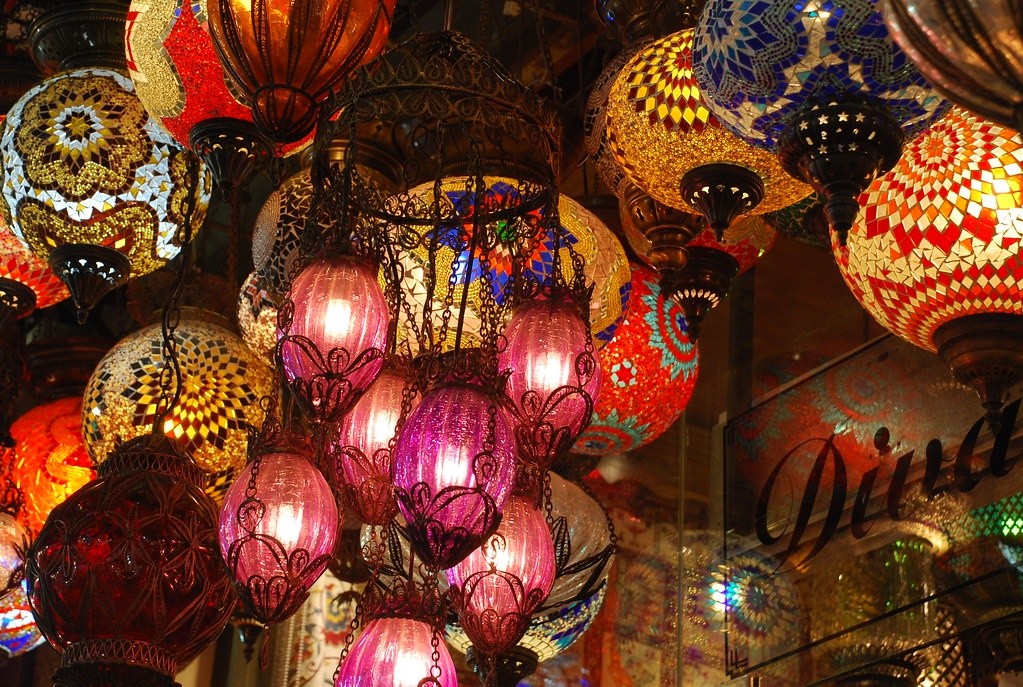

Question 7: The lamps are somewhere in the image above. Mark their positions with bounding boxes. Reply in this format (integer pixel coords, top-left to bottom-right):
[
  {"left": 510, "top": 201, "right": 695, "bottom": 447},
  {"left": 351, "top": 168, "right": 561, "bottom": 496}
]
[{"left": 0, "top": 0, "right": 1023, "bottom": 687}]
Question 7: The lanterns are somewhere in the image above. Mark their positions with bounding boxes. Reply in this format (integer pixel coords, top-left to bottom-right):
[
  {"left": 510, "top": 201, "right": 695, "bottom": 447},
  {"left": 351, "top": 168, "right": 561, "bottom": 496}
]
[{"left": 1, "top": 0, "right": 1023, "bottom": 687}]
